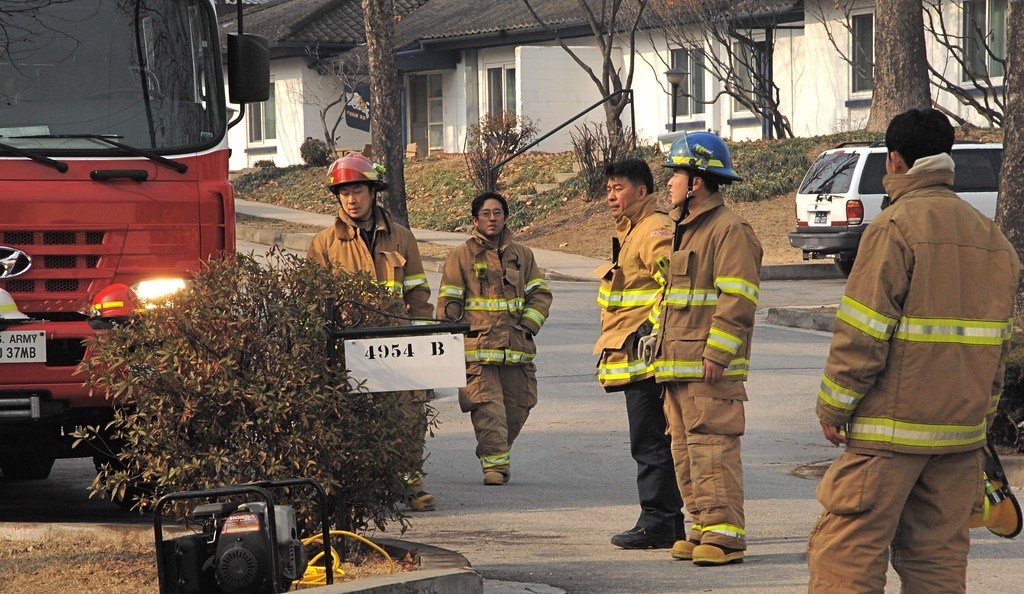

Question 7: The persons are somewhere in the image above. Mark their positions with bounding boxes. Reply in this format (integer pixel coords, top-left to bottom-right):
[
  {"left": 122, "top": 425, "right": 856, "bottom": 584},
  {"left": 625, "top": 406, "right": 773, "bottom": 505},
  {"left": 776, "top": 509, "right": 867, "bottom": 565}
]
[
  {"left": 593, "top": 131, "right": 764, "bottom": 565},
  {"left": 804, "top": 109, "right": 1020, "bottom": 594},
  {"left": 308, "top": 157, "right": 435, "bottom": 512},
  {"left": 436, "top": 193, "right": 553, "bottom": 485}
]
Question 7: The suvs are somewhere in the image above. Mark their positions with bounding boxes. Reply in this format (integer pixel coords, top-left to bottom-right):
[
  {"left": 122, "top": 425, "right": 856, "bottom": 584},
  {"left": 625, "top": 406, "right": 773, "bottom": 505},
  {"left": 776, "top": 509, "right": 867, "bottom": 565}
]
[{"left": 788, "top": 139, "right": 1004, "bottom": 281}]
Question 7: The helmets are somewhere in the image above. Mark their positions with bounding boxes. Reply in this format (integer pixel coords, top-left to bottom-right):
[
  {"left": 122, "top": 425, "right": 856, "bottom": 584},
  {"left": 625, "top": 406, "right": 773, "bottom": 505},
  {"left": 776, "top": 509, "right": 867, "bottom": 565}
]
[
  {"left": 326, "top": 153, "right": 385, "bottom": 195},
  {"left": 661, "top": 130, "right": 744, "bottom": 181},
  {"left": 967, "top": 448, "right": 1021, "bottom": 538}
]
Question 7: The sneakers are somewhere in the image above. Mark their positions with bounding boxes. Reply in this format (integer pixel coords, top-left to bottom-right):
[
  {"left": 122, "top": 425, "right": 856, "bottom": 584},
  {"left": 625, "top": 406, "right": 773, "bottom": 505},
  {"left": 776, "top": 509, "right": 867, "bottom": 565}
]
[{"left": 612, "top": 525, "right": 686, "bottom": 549}]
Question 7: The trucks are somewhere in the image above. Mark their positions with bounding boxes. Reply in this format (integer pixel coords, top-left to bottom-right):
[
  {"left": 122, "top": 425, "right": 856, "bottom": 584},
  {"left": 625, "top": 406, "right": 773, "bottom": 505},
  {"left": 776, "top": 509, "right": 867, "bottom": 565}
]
[{"left": 0, "top": 0, "right": 273, "bottom": 519}]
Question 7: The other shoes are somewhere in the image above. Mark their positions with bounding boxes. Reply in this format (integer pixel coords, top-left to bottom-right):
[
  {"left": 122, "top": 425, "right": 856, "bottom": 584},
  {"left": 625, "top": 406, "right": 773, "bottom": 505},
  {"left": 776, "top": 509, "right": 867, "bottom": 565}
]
[
  {"left": 484, "top": 472, "right": 511, "bottom": 485},
  {"left": 693, "top": 544, "right": 744, "bottom": 563},
  {"left": 406, "top": 491, "right": 436, "bottom": 510},
  {"left": 671, "top": 540, "right": 701, "bottom": 560}
]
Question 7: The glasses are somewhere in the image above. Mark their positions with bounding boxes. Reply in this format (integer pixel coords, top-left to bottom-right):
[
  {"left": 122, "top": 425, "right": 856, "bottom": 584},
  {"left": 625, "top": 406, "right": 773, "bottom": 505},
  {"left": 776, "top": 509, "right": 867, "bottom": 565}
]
[{"left": 476, "top": 209, "right": 506, "bottom": 218}]
